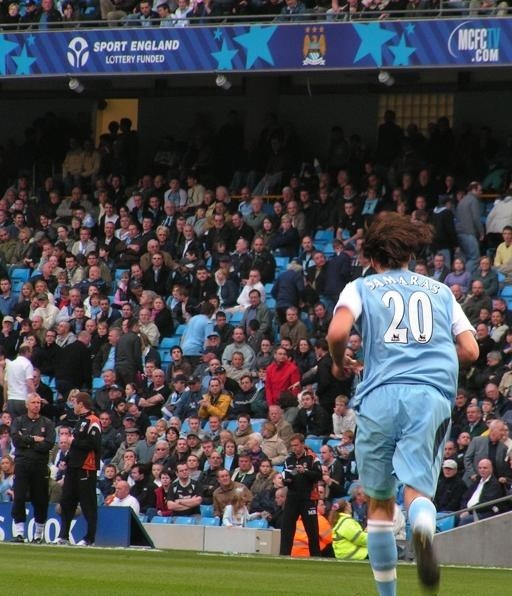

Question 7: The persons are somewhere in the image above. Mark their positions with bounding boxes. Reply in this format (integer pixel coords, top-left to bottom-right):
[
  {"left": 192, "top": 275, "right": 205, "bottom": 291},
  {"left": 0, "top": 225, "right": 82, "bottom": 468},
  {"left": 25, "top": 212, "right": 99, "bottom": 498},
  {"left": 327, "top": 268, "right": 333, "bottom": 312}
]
[
  {"left": 0, "top": 103, "right": 512, "bottom": 596},
  {"left": 0, "top": 0, "right": 512, "bottom": 29}
]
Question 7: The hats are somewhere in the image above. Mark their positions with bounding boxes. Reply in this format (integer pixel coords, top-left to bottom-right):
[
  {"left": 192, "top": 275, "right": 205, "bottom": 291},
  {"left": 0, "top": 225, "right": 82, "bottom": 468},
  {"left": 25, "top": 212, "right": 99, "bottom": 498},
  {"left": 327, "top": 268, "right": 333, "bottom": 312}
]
[
  {"left": 121, "top": 412, "right": 137, "bottom": 422},
  {"left": 186, "top": 431, "right": 201, "bottom": 440},
  {"left": 125, "top": 428, "right": 140, "bottom": 434},
  {"left": 129, "top": 280, "right": 145, "bottom": 289},
  {"left": 103, "top": 384, "right": 124, "bottom": 392},
  {"left": 264, "top": 422, "right": 277, "bottom": 433},
  {"left": 3, "top": 315, "right": 14, "bottom": 323},
  {"left": 207, "top": 332, "right": 220, "bottom": 338},
  {"left": 38, "top": 293, "right": 48, "bottom": 301},
  {"left": 184, "top": 376, "right": 200, "bottom": 383},
  {"left": 216, "top": 367, "right": 226, "bottom": 373},
  {"left": 171, "top": 374, "right": 186, "bottom": 382},
  {"left": 442, "top": 459, "right": 457, "bottom": 469}
]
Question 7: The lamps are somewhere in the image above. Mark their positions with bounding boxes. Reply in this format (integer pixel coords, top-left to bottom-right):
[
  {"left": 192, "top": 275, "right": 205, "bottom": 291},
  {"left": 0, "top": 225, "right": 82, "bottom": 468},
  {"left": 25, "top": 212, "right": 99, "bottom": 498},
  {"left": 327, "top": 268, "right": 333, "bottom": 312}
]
[
  {"left": 68, "top": 74, "right": 84, "bottom": 93},
  {"left": 216, "top": 73, "right": 231, "bottom": 90},
  {"left": 378, "top": 65, "right": 395, "bottom": 86}
]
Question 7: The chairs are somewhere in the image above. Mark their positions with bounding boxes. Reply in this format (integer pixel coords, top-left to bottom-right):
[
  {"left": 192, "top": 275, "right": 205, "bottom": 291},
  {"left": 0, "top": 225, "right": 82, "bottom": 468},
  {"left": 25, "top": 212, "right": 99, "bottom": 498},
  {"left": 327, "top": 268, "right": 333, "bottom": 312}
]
[
  {"left": 305, "top": 438, "right": 323, "bottom": 463},
  {"left": 10, "top": 269, "right": 42, "bottom": 293},
  {"left": 92, "top": 378, "right": 104, "bottom": 398},
  {"left": 313, "top": 230, "right": 355, "bottom": 256},
  {"left": 107, "top": 268, "right": 131, "bottom": 304},
  {"left": 327, "top": 439, "right": 341, "bottom": 447},
  {"left": 151, "top": 418, "right": 267, "bottom": 433},
  {"left": 436, "top": 512, "right": 455, "bottom": 532},
  {"left": 139, "top": 505, "right": 271, "bottom": 531},
  {"left": 272, "top": 465, "right": 285, "bottom": 472},
  {"left": 41, "top": 375, "right": 57, "bottom": 400},
  {"left": 159, "top": 324, "right": 188, "bottom": 368},
  {"left": 497, "top": 272, "right": 512, "bottom": 311},
  {"left": 226, "top": 256, "right": 290, "bottom": 346}
]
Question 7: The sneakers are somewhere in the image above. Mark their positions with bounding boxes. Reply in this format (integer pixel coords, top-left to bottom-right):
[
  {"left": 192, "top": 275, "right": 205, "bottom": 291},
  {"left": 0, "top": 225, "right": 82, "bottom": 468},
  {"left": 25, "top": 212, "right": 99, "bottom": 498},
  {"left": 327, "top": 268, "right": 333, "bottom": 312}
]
[
  {"left": 10, "top": 535, "right": 24, "bottom": 543},
  {"left": 51, "top": 537, "right": 71, "bottom": 544},
  {"left": 30, "top": 537, "right": 44, "bottom": 543},
  {"left": 76, "top": 538, "right": 95, "bottom": 546},
  {"left": 411, "top": 527, "right": 440, "bottom": 588}
]
